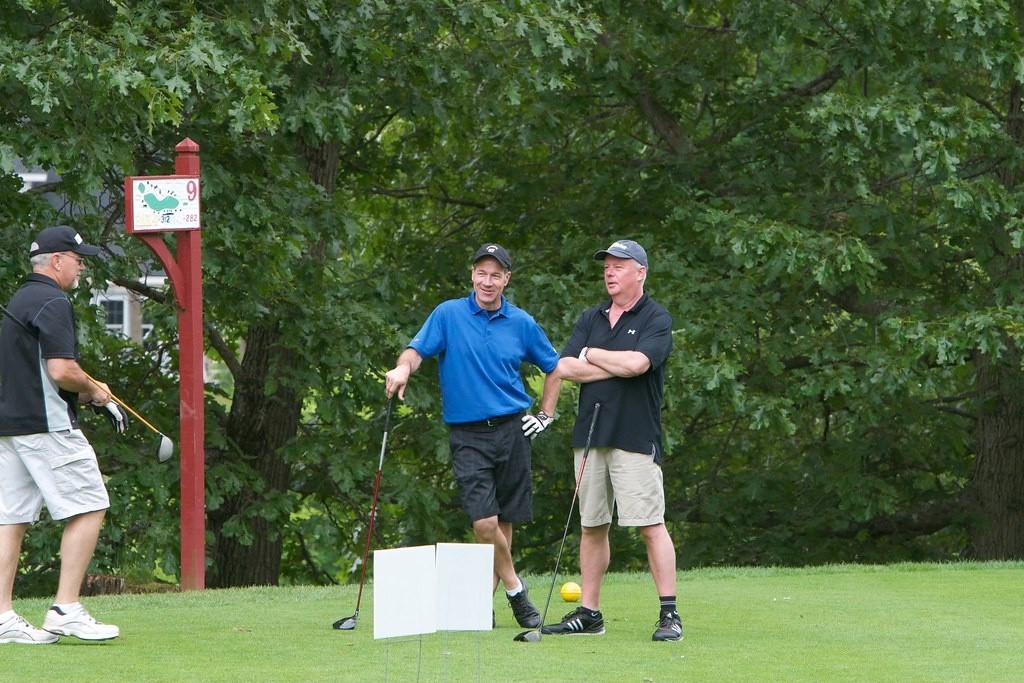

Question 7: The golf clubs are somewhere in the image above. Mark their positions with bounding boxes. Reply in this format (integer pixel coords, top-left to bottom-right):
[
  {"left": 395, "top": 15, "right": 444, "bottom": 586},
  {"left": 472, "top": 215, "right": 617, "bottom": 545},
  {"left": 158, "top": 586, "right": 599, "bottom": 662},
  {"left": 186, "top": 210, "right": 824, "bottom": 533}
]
[
  {"left": 331, "top": 394, "right": 394, "bottom": 631},
  {"left": 82, "top": 368, "right": 174, "bottom": 463},
  {"left": 511, "top": 402, "right": 601, "bottom": 643}
]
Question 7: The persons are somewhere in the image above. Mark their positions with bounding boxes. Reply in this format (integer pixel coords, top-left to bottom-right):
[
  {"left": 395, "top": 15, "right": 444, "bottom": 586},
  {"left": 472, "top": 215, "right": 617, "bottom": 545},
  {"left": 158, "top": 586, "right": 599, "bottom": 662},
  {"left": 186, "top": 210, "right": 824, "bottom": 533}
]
[
  {"left": 0, "top": 226, "right": 119, "bottom": 646},
  {"left": 541, "top": 241, "right": 683, "bottom": 641},
  {"left": 385, "top": 244, "right": 561, "bottom": 627}
]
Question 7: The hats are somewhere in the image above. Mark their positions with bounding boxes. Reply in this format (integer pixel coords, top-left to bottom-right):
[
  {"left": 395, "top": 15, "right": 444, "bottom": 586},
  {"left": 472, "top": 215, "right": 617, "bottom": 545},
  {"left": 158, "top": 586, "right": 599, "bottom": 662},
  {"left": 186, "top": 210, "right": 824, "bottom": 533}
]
[
  {"left": 593, "top": 240, "right": 648, "bottom": 269},
  {"left": 473, "top": 243, "right": 512, "bottom": 269},
  {"left": 30, "top": 226, "right": 101, "bottom": 258}
]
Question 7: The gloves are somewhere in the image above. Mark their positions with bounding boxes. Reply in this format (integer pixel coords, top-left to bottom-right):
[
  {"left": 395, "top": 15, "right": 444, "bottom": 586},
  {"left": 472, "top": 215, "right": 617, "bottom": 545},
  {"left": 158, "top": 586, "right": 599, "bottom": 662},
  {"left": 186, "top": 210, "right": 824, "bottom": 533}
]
[
  {"left": 86, "top": 398, "right": 129, "bottom": 433},
  {"left": 522, "top": 410, "right": 555, "bottom": 440},
  {"left": 578, "top": 347, "right": 589, "bottom": 363}
]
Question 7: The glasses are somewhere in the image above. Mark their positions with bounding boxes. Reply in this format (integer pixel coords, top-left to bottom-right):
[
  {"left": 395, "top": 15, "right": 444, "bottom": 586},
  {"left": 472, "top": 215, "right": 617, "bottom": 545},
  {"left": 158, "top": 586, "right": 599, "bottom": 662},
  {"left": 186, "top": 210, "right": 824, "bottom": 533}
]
[{"left": 60, "top": 252, "right": 84, "bottom": 266}]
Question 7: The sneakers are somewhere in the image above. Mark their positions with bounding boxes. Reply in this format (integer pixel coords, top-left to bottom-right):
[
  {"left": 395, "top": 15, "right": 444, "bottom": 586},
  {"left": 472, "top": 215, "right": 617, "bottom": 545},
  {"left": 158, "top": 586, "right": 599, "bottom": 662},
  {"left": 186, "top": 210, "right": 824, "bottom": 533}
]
[
  {"left": 540, "top": 607, "right": 606, "bottom": 635},
  {"left": 506, "top": 576, "right": 541, "bottom": 628},
  {"left": 0, "top": 614, "right": 60, "bottom": 644},
  {"left": 492, "top": 610, "right": 496, "bottom": 629},
  {"left": 42, "top": 605, "right": 120, "bottom": 641},
  {"left": 652, "top": 610, "right": 683, "bottom": 641}
]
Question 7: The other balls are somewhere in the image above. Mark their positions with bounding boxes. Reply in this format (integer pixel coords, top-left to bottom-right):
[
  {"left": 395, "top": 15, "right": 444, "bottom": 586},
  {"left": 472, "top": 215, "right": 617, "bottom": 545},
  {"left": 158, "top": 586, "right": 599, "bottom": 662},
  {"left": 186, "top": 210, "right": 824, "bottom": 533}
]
[{"left": 560, "top": 581, "right": 582, "bottom": 602}]
